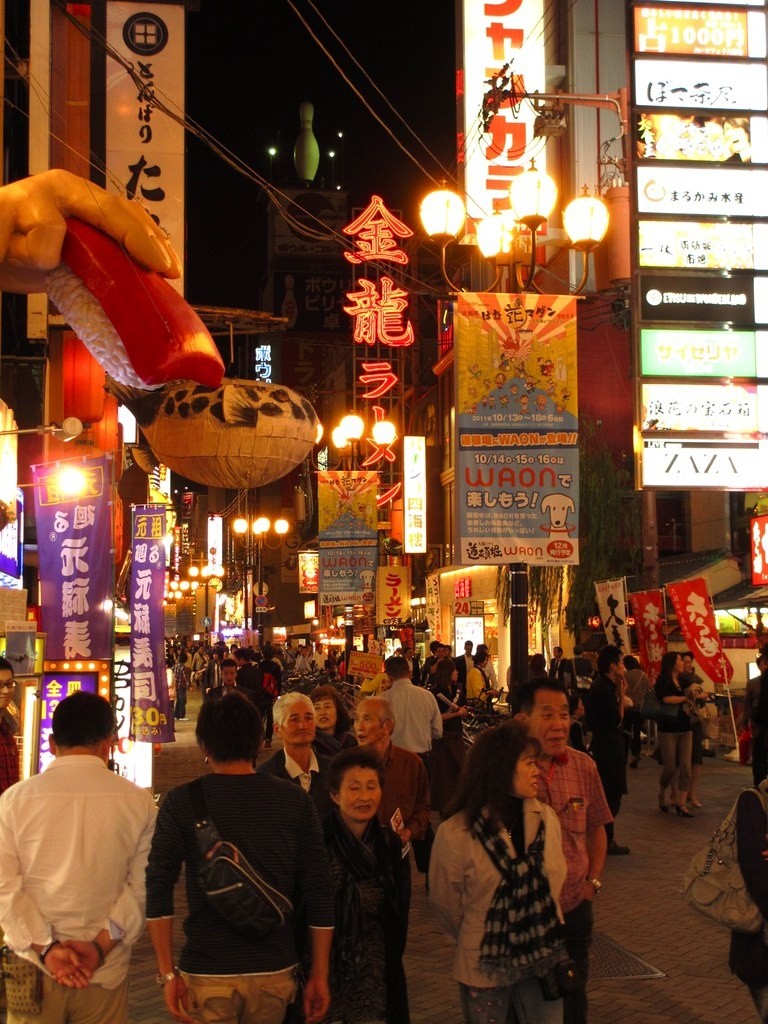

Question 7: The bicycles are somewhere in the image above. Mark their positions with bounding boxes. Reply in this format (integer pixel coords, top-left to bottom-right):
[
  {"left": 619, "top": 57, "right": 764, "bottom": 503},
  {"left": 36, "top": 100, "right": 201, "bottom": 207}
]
[
  {"left": 460, "top": 685, "right": 505, "bottom": 752},
  {"left": 278, "top": 669, "right": 352, "bottom": 714}
]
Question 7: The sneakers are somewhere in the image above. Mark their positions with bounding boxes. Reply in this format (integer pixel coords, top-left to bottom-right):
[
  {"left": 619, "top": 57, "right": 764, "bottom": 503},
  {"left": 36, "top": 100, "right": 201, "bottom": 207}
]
[
  {"left": 179, "top": 716, "right": 191, "bottom": 720},
  {"left": 264, "top": 743, "right": 272, "bottom": 750}
]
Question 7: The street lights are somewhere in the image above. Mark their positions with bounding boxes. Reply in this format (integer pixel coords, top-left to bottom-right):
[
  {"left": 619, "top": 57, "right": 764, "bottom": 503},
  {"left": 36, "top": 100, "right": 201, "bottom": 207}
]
[
  {"left": 233, "top": 516, "right": 290, "bottom": 652},
  {"left": 188, "top": 564, "right": 224, "bottom": 650},
  {"left": 419, "top": 162, "right": 611, "bottom": 720}
]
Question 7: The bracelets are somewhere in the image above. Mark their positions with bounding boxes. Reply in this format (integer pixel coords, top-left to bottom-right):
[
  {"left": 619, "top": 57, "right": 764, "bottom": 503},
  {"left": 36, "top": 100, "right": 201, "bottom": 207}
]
[
  {"left": 40, "top": 939, "right": 60, "bottom": 964},
  {"left": 585, "top": 877, "right": 602, "bottom": 894},
  {"left": 156, "top": 968, "right": 179, "bottom": 985},
  {"left": 94, "top": 941, "right": 105, "bottom": 965}
]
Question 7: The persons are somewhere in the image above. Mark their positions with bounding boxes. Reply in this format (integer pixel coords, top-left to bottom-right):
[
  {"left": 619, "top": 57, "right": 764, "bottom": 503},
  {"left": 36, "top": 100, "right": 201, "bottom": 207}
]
[
  {"left": 622, "top": 654, "right": 650, "bottom": 769},
  {"left": 1, "top": 656, "right": 336, "bottom": 1024},
  {"left": 568, "top": 696, "right": 586, "bottom": 752},
  {"left": 327, "top": 697, "right": 431, "bottom": 955},
  {"left": 262, "top": 641, "right": 333, "bottom": 742},
  {"left": 567, "top": 644, "right": 593, "bottom": 693},
  {"left": 679, "top": 652, "right": 704, "bottom": 808},
  {"left": 654, "top": 652, "right": 695, "bottom": 817},
  {"left": 379, "top": 641, "right": 501, "bottom": 890},
  {"left": 583, "top": 645, "right": 631, "bottom": 854},
  {"left": 735, "top": 644, "right": 768, "bottom": 787},
  {"left": 427, "top": 718, "right": 568, "bottom": 1024},
  {"left": 506, "top": 646, "right": 567, "bottom": 693},
  {"left": 164, "top": 636, "right": 191, "bottom": 721},
  {"left": 511, "top": 675, "right": 616, "bottom": 1024},
  {"left": 191, "top": 641, "right": 264, "bottom": 698},
  {"left": 295, "top": 746, "right": 414, "bottom": 1024}
]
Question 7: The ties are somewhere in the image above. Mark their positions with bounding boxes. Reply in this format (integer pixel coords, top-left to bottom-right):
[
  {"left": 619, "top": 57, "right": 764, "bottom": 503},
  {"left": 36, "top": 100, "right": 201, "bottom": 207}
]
[
  {"left": 216, "top": 661, "right": 221, "bottom": 686},
  {"left": 556, "top": 660, "right": 559, "bottom": 668}
]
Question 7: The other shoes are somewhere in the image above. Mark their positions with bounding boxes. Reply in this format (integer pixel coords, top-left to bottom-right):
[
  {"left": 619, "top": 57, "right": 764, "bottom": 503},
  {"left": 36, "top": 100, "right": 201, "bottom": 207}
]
[
  {"left": 629, "top": 755, "right": 642, "bottom": 769},
  {"left": 607, "top": 839, "right": 631, "bottom": 857},
  {"left": 657, "top": 792, "right": 704, "bottom": 818}
]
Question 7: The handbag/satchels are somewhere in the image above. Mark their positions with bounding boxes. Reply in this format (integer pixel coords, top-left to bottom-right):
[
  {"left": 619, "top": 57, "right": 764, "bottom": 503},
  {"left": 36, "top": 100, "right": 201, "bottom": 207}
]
[
  {"left": 262, "top": 673, "right": 280, "bottom": 698},
  {"left": 537, "top": 958, "right": 586, "bottom": 1001},
  {"left": 3, "top": 948, "right": 44, "bottom": 1015},
  {"left": 640, "top": 675, "right": 682, "bottom": 720},
  {"left": 623, "top": 694, "right": 634, "bottom": 708},
  {"left": 194, "top": 781, "right": 295, "bottom": 941},
  {"left": 684, "top": 788, "right": 765, "bottom": 934}
]
